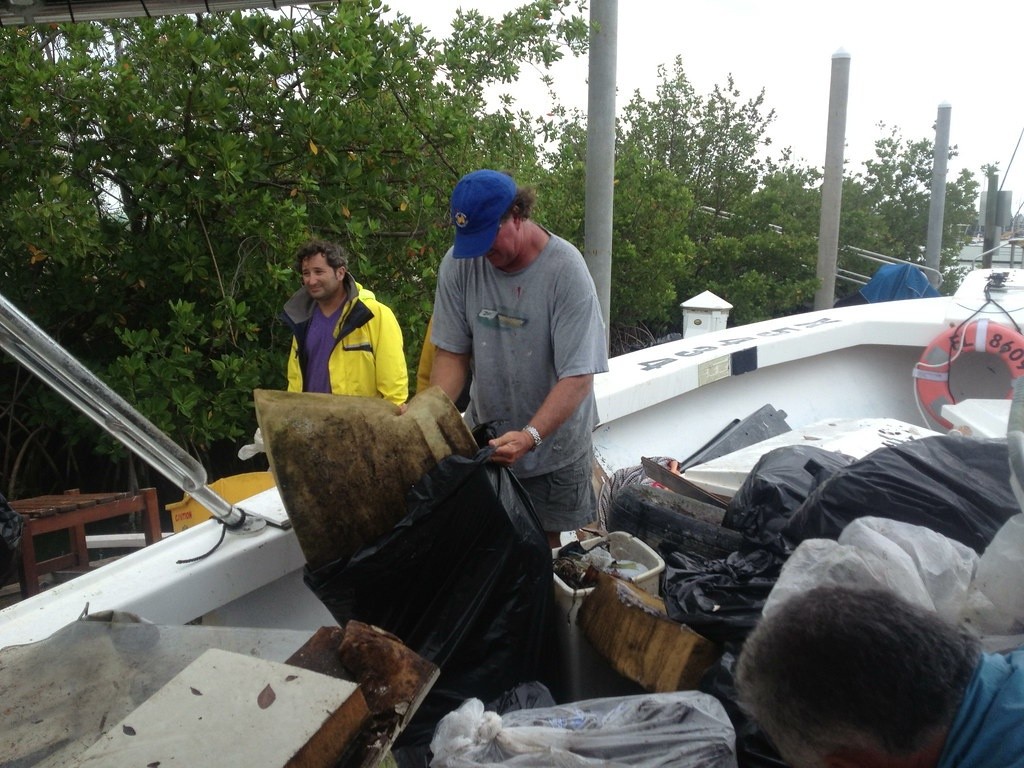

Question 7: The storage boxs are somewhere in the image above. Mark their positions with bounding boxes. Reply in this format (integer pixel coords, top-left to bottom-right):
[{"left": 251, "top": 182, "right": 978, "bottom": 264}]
[
  {"left": 550, "top": 531, "right": 666, "bottom": 704},
  {"left": 577, "top": 573, "right": 716, "bottom": 692}
]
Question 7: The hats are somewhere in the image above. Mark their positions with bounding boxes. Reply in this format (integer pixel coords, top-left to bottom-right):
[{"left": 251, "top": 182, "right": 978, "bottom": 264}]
[{"left": 451, "top": 170, "right": 517, "bottom": 258}]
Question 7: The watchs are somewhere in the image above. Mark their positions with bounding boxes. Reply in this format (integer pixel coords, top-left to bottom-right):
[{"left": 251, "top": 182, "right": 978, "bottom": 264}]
[{"left": 522, "top": 425, "right": 543, "bottom": 454}]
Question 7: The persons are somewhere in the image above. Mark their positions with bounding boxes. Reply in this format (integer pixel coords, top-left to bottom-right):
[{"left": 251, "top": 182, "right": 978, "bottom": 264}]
[
  {"left": 415, "top": 309, "right": 472, "bottom": 417},
  {"left": 427, "top": 168, "right": 610, "bottom": 548},
  {"left": 732, "top": 584, "right": 1024, "bottom": 768},
  {"left": 283, "top": 239, "right": 410, "bottom": 413}
]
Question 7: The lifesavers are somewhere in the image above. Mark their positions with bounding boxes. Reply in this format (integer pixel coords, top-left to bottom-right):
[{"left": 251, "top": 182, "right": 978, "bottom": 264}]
[{"left": 912, "top": 318, "right": 1024, "bottom": 438}]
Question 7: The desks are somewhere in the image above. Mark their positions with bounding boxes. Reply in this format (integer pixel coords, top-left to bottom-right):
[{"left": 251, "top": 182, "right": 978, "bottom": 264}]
[{"left": 0, "top": 487, "right": 162, "bottom": 601}]
